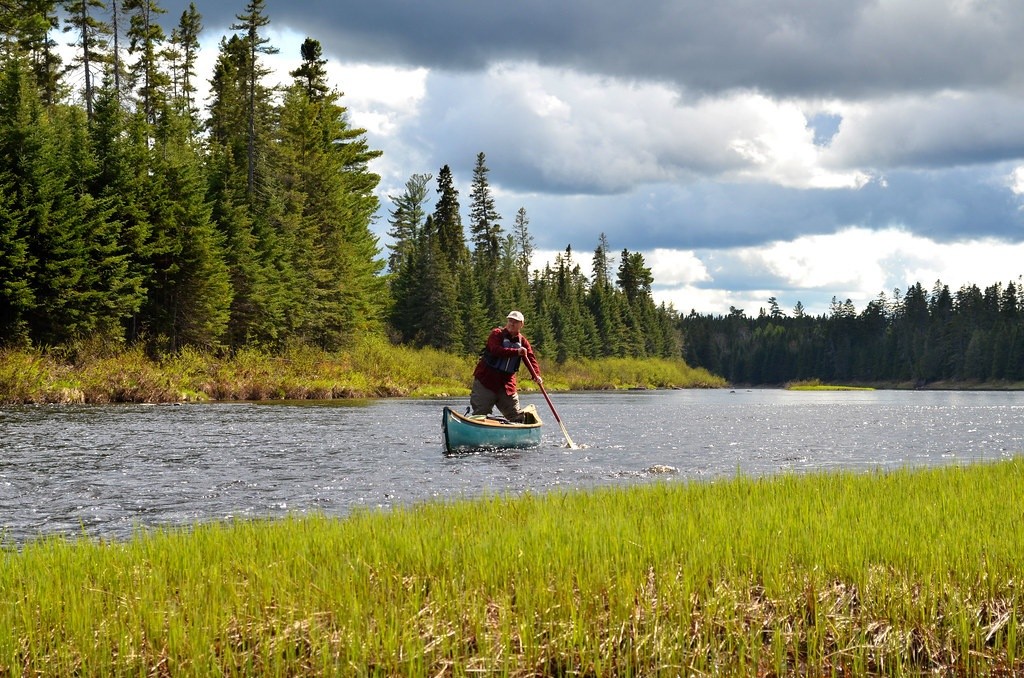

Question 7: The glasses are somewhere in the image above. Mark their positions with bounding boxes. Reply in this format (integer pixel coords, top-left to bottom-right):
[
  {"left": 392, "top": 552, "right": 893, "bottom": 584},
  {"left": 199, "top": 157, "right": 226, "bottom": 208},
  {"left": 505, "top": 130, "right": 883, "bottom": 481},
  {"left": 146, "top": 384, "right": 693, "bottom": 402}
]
[{"left": 508, "top": 319, "right": 521, "bottom": 323}]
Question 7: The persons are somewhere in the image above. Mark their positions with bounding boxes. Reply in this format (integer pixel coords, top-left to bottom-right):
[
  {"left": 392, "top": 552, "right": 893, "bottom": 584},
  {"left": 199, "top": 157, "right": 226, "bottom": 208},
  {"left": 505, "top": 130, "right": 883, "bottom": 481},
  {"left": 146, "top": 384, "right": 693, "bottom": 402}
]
[{"left": 470, "top": 310, "right": 543, "bottom": 424}]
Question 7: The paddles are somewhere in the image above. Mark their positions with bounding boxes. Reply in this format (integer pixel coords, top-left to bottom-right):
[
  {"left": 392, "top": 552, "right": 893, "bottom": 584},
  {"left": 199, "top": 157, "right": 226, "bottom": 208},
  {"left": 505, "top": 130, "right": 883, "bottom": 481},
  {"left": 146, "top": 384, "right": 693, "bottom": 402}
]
[{"left": 522, "top": 356, "right": 576, "bottom": 448}]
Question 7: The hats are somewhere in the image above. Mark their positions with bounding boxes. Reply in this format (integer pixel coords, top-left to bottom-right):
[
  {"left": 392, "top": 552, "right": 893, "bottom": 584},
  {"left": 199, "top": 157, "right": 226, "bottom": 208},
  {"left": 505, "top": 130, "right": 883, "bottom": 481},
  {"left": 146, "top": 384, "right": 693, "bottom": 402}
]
[{"left": 506, "top": 311, "right": 524, "bottom": 325}]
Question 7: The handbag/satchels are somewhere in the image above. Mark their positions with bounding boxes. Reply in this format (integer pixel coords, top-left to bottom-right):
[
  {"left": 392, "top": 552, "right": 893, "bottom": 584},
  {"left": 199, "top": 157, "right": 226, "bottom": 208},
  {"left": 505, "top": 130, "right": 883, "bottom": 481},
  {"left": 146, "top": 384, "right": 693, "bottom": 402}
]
[{"left": 518, "top": 404, "right": 540, "bottom": 424}]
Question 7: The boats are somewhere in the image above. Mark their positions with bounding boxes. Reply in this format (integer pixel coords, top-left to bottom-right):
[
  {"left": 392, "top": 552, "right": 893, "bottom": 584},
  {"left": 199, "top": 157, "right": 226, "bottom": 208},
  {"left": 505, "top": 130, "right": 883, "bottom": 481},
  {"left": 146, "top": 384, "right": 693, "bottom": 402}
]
[{"left": 441, "top": 402, "right": 543, "bottom": 452}]
